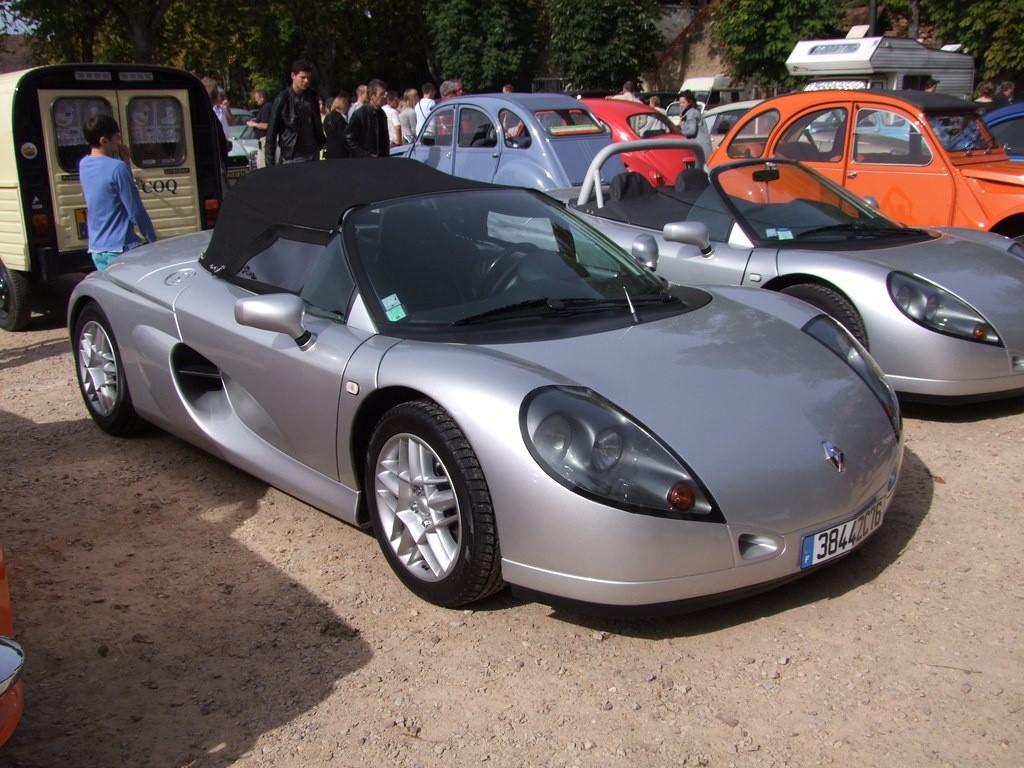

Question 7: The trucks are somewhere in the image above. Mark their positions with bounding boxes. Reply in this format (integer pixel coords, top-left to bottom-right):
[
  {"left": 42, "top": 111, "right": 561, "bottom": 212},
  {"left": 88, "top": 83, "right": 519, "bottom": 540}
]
[
  {"left": 785, "top": 24, "right": 976, "bottom": 143},
  {"left": 0, "top": 62, "right": 227, "bottom": 332}
]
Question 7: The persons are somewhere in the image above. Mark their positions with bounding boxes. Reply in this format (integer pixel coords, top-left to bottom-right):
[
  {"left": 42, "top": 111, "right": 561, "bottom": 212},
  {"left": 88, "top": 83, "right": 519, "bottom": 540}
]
[
  {"left": 78, "top": 113, "right": 158, "bottom": 271},
  {"left": 199, "top": 70, "right": 273, "bottom": 200},
  {"left": 265, "top": 59, "right": 528, "bottom": 167},
  {"left": 676, "top": 89, "right": 714, "bottom": 162},
  {"left": 923, "top": 79, "right": 1015, "bottom": 110},
  {"left": 608, "top": 82, "right": 670, "bottom": 138}
]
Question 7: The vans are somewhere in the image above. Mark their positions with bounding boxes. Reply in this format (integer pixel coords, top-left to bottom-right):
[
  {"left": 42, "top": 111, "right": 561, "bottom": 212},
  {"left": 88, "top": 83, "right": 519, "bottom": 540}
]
[{"left": 680, "top": 75, "right": 764, "bottom": 113}]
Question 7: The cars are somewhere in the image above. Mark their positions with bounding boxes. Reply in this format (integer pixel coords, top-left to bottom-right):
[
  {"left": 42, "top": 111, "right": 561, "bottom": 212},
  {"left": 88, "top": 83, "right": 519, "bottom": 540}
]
[
  {"left": 66, "top": 150, "right": 904, "bottom": 610},
  {"left": 566, "top": 140, "right": 1024, "bottom": 410},
  {"left": 228, "top": 109, "right": 257, "bottom": 185},
  {"left": 706, "top": 89, "right": 1024, "bottom": 238},
  {"left": 663, "top": 102, "right": 1024, "bottom": 160},
  {"left": 390, "top": 91, "right": 628, "bottom": 191},
  {"left": 505, "top": 97, "right": 698, "bottom": 189}
]
